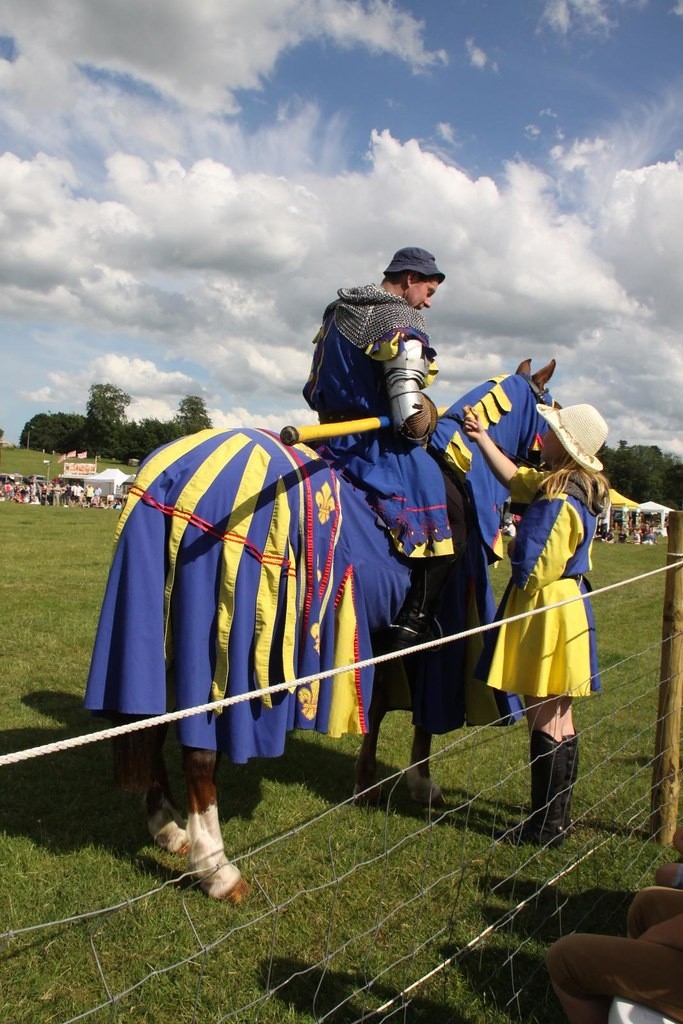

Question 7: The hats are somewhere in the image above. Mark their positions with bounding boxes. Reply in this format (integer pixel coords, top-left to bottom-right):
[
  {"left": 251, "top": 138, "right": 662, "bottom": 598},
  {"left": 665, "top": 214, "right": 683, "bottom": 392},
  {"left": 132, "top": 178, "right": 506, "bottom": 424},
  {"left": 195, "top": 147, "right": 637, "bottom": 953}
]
[
  {"left": 536, "top": 403, "right": 608, "bottom": 472},
  {"left": 383, "top": 247, "right": 446, "bottom": 284}
]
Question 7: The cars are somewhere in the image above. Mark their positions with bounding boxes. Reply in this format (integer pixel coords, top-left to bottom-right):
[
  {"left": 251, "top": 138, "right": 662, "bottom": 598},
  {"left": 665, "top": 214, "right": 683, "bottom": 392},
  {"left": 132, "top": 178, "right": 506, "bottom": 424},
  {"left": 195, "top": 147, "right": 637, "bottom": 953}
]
[{"left": 27, "top": 474, "right": 45, "bottom": 486}]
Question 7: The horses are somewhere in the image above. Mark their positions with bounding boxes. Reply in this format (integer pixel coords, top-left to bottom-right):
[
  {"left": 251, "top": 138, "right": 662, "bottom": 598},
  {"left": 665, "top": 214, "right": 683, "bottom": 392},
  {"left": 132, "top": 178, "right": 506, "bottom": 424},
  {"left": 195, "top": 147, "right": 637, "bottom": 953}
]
[{"left": 80, "top": 358, "right": 559, "bottom": 908}]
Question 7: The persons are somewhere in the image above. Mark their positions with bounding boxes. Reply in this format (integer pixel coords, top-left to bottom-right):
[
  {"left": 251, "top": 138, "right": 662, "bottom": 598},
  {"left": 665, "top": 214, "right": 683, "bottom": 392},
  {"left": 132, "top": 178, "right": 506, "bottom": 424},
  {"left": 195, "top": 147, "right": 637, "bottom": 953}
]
[
  {"left": 560, "top": 831, "right": 683, "bottom": 1024},
  {"left": 300, "top": 247, "right": 456, "bottom": 643},
  {"left": 463, "top": 401, "right": 602, "bottom": 845},
  {"left": 0, "top": 476, "right": 131, "bottom": 509},
  {"left": 594, "top": 518, "right": 657, "bottom": 545}
]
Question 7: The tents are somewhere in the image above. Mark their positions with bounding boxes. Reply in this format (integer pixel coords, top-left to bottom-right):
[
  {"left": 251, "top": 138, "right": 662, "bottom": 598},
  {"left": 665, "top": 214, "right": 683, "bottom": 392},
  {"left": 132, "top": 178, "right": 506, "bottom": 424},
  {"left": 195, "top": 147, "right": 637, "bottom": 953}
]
[
  {"left": 88, "top": 467, "right": 131, "bottom": 491},
  {"left": 593, "top": 488, "right": 675, "bottom": 533}
]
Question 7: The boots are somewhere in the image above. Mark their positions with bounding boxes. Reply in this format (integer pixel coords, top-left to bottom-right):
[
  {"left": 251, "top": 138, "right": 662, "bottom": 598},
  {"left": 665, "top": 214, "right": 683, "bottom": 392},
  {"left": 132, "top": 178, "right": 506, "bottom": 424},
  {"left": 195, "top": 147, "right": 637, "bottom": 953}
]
[
  {"left": 512, "top": 733, "right": 579, "bottom": 837},
  {"left": 382, "top": 540, "right": 464, "bottom": 650},
  {"left": 494, "top": 729, "right": 563, "bottom": 848}
]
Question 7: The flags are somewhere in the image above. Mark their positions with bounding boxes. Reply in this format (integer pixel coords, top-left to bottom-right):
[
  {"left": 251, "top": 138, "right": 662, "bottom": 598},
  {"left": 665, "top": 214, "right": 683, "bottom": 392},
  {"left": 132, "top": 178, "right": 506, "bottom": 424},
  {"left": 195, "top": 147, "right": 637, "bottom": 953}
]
[{"left": 57, "top": 450, "right": 88, "bottom": 463}]
